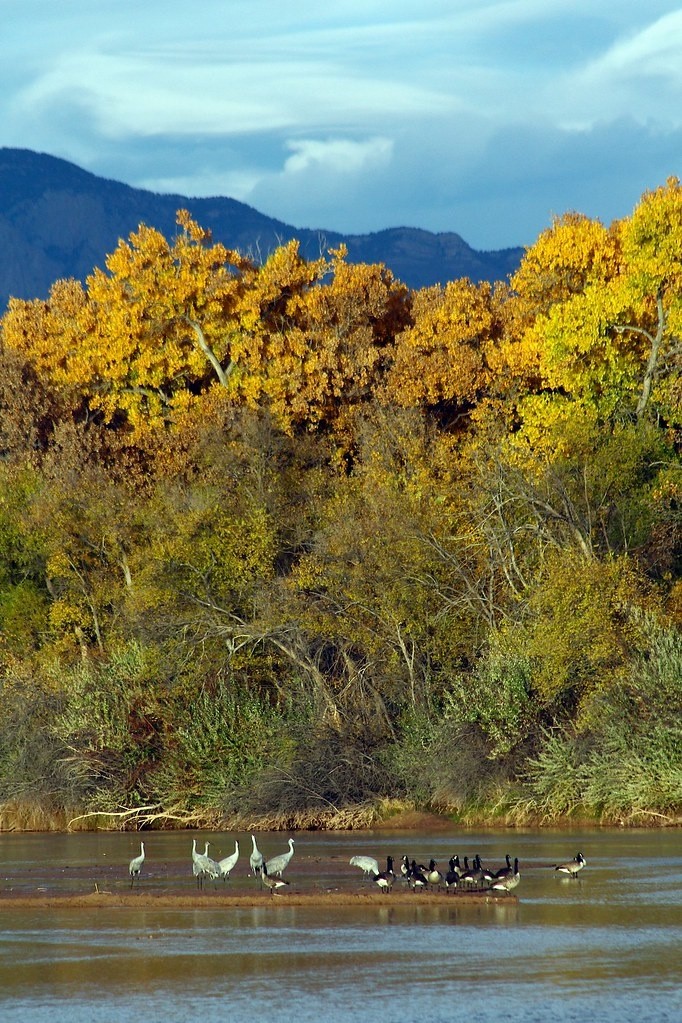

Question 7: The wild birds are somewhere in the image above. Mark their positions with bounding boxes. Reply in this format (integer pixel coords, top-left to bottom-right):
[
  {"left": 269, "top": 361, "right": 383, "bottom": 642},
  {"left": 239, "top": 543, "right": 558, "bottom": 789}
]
[
  {"left": 129, "top": 841, "right": 146, "bottom": 890},
  {"left": 554, "top": 852, "right": 587, "bottom": 879},
  {"left": 349, "top": 847, "right": 521, "bottom": 896},
  {"left": 191, "top": 833, "right": 295, "bottom": 894}
]
